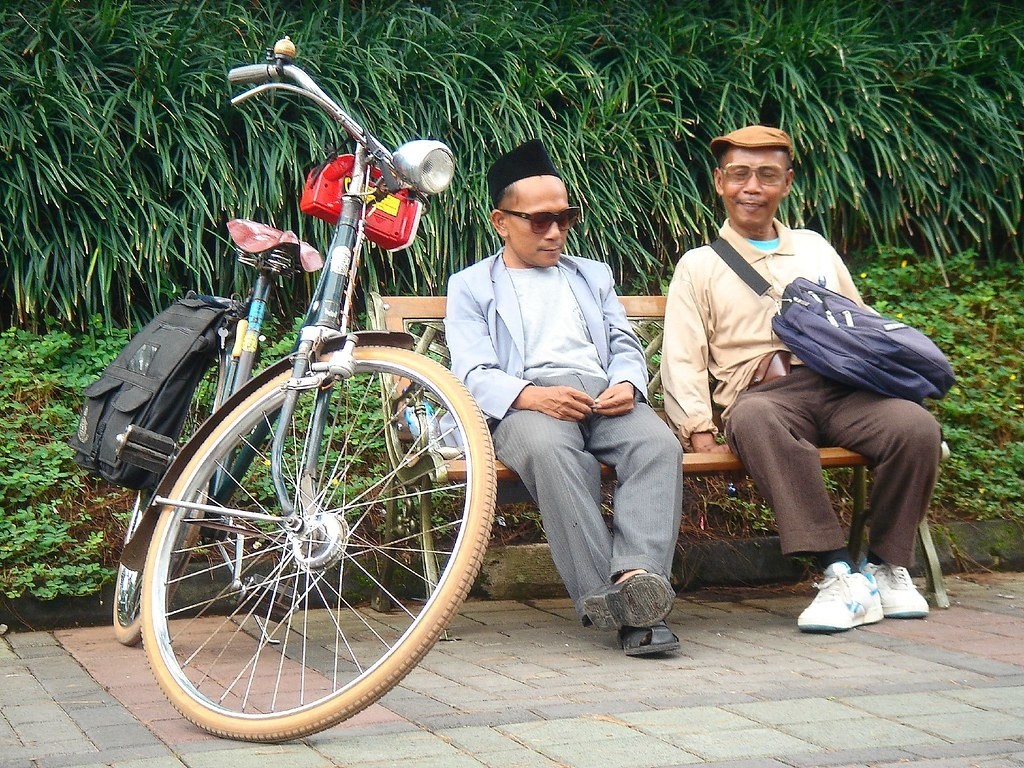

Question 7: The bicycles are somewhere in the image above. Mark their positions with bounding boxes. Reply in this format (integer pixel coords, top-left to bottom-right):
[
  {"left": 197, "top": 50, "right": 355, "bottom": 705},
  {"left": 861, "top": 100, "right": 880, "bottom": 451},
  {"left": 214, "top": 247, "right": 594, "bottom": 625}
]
[{"left": 110, "top": 39, "right": 497, "bottom": 743}]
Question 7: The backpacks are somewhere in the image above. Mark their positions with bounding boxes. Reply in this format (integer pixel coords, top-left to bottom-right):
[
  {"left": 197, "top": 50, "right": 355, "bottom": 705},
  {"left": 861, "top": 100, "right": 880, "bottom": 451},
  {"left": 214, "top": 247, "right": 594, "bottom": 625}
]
[{"left": 68, "top": 298, "right": 230, "bottom": 491}]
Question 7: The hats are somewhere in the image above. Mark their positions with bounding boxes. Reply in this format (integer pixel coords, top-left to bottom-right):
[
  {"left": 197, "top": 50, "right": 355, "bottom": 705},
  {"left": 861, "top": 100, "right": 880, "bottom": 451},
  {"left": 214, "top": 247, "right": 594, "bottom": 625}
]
[
  {"left": 710, "top": 125, "right": 794, "bottom": 161},
  {"left": 487, "top": 139, "right": 562, "bottom": 199}
]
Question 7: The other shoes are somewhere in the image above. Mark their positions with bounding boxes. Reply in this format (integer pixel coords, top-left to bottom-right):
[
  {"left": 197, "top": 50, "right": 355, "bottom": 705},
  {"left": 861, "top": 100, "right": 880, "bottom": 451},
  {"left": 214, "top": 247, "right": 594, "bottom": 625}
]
[
  {"left": 583, "top": 573, "right": 676, "bottom": 633},
  {"left": 617, "top": 620, "right": 681, "bottom": 655}
]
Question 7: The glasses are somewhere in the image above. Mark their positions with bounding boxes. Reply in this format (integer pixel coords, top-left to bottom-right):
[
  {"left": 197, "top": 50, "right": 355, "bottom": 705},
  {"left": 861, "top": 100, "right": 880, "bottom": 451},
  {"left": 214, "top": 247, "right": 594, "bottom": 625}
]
[
  {"left": 498, "top": 206, "right": 580, "bottom": 235},
  {"left": 718, "top": 162, "right": 788, "bottom": 186}
]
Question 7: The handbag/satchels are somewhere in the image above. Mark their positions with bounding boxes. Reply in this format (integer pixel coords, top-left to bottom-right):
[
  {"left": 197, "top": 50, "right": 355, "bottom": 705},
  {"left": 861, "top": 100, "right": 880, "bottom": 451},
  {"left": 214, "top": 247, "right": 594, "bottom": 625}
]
[{"left": 710, "top": 237, "right": 956, "bottom": 405}]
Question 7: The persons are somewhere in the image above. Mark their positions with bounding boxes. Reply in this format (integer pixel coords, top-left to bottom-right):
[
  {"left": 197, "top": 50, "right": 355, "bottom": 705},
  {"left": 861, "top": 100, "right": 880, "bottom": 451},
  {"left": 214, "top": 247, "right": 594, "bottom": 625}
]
[
  {"left": 660, "top": 125, "right": 940, "bottom": 633},
  {"left": 439, "top": 139, "right": 685, "bottom": 655}
]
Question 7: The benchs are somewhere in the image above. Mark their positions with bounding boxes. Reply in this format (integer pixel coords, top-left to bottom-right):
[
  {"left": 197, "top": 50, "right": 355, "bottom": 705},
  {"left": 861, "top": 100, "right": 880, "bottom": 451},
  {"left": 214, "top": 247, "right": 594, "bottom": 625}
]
[{"left": 365, "top": 292, "right": 952, "bottom": 640}]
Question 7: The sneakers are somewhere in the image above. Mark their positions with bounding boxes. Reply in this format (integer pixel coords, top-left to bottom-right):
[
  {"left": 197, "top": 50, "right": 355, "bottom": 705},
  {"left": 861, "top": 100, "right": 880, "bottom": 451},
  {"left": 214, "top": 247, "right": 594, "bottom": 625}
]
[
  {"left": 798, "top": 560, "right": 884, "bottom": 631},
  {"left": 857, "top": 557, "right": 929, "bottom": 617}
]
